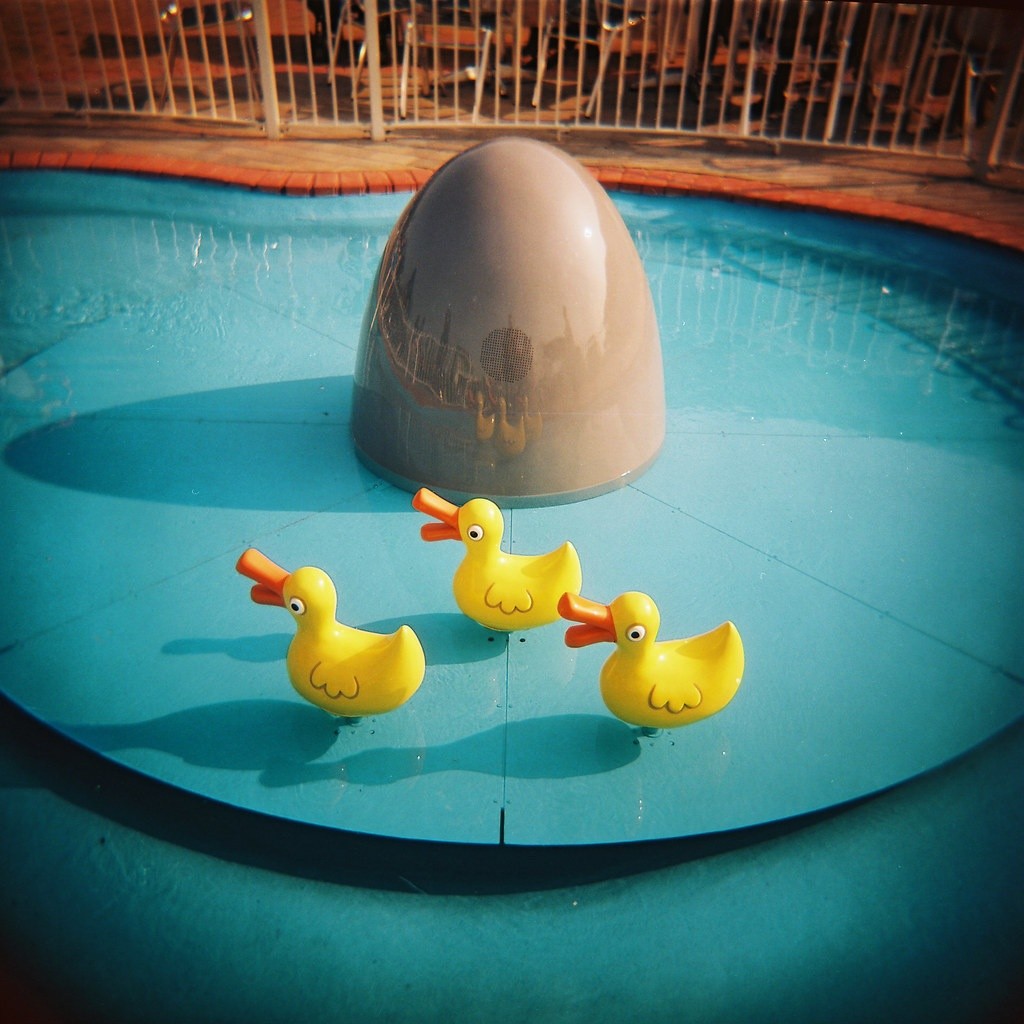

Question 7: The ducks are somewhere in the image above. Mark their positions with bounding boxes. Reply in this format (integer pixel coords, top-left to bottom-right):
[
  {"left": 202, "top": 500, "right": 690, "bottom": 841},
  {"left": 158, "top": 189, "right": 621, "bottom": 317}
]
[
  {"left": 236, "top": 549, "right": 425, "bottom": 716},
  {"left": 558, "top": 590, "right": 745, "bottom": 729},
  {"left": 412, "top": 487, "right": 582, "bottom": 631}
]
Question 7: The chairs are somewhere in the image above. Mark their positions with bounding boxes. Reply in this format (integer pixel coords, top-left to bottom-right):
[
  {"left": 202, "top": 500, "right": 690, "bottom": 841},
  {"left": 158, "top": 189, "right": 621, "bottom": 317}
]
[
  {"left": 327, "top": 0, "right": 416, "bottom": 102},
  {"left": 897, "top": 0, "right": 1014, "bottom": 152},
  {"left": 157, "top": 0, "right": 264, "bottom": 116},
  {"left": 530, "top": 0, "right": 659, "bottom": 120},
  {"left": 400, "top": 0, "right": 507, "bottom": 123}
]
